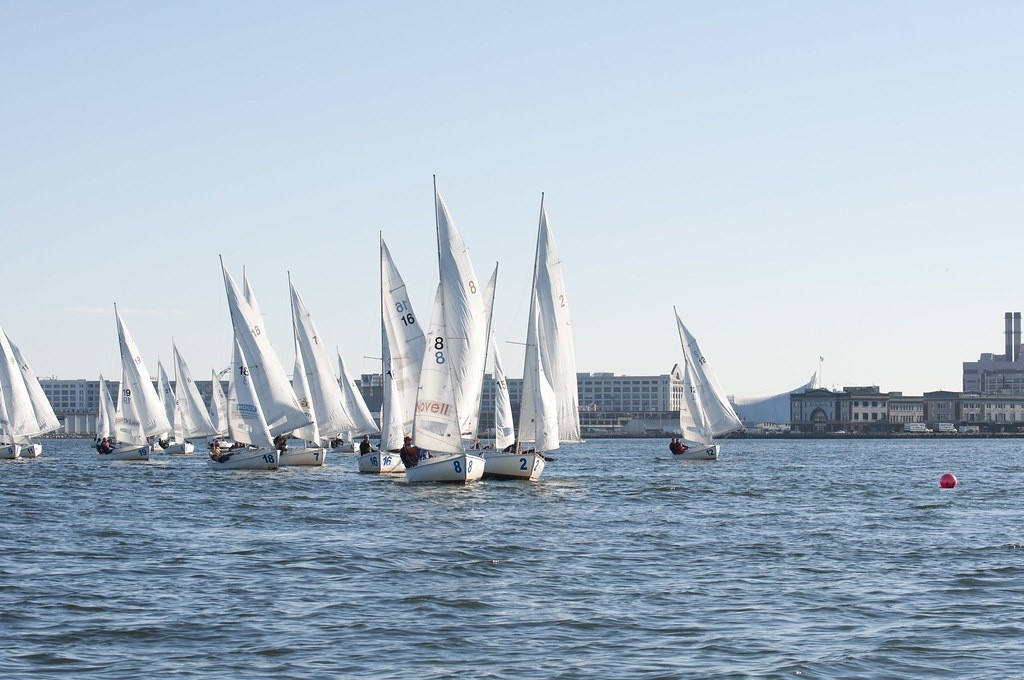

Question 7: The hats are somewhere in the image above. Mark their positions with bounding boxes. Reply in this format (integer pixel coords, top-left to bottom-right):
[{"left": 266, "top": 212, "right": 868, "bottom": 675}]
[{"left": 404, "top": 436, "right": 412, "bottom": 441}]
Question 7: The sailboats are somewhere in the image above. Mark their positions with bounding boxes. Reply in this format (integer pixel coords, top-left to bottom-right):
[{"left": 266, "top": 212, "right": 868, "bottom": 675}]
[
  {"left": 463, "top": 191, "right": 586, "bottom": 479},
  {"left": 672, "top": 305, "right": 745, "bottom": 460},
  {"left": 404, "top": 174, "right": 487, "bottom": 482},
  {"left": 356, "top": 230, "right": 428, "bottom": 473},
  {"left": 0, "top": 253, "right": 382, "bottom": 471}
]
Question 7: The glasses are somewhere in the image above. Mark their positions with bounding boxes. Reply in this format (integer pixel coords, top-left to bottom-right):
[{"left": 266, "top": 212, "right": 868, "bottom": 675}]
[{"left": 364, "top": 436, "right": 369, "bottom": 438}]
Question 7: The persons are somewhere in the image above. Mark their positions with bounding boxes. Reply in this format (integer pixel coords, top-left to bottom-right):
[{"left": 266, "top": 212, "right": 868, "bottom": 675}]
[
  {"left": 359, "top": 434, "right": 371, "bottom": 456},
  {"left": 669, "top": 438, "right": 687, "bottom": 455},
  {"left": 503, "top": 437, "right": 522, "bottom": 455},
  {"left": 473, "top": 438, "right": 494, "bottom": 449},
  {"left": 331, "top": 437, "right": 344, "bottom": 449},
  {"left": 274, "top": 435, "right": 287, "bottom": 456},
  {"left": 400, "top": 436, "right": 433, "bottom": 468},
  {"left": 96, "top": 437, "right": 113, "bottom": 454},
  {"left": 159, "top": 438, "right": 169, "bottom": 448},
  {"left": 211, "top": 440, "right": 223, "bottom": 462}
]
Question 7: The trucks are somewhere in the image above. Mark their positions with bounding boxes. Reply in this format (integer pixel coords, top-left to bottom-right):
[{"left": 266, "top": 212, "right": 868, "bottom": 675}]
[
  {"left": 932, "top": 422, "right": 958, "bottom": 433},
  {"left": 904, "top": 422, "right": 930, "bottom": 433}
]
